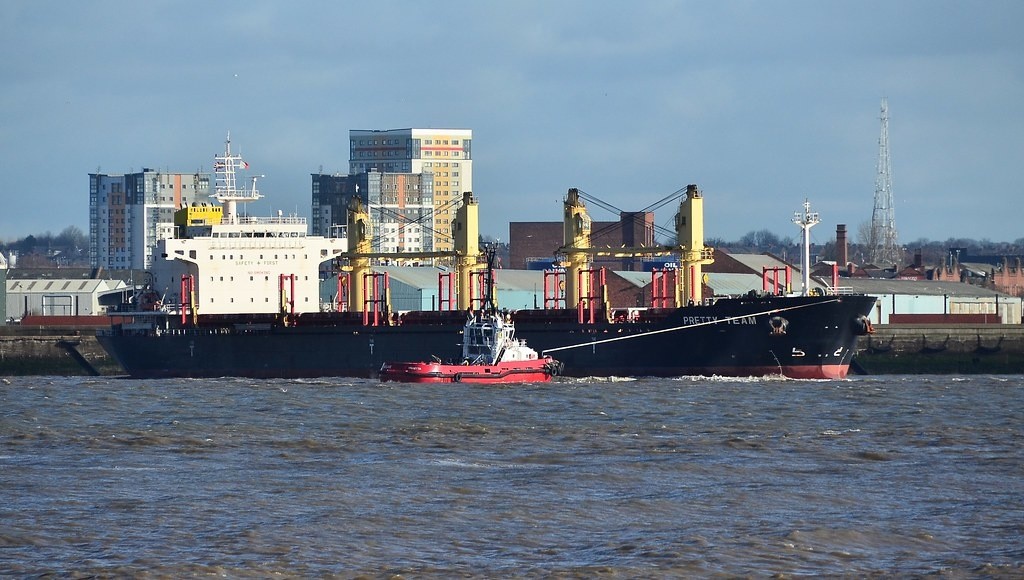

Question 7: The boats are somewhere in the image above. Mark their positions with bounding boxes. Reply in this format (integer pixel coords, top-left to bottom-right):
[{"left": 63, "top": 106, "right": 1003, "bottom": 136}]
[
  {"left": 379, "top": 238, "right": 564, "bottom": 384},
  {"left": 91, "top": 130, "right": 878, "bottom": 379}
]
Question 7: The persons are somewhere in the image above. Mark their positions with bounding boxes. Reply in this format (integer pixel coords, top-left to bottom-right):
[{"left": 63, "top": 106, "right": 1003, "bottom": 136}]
[{"left": 155, "top": 325, "right": 160, "bottom": 337}]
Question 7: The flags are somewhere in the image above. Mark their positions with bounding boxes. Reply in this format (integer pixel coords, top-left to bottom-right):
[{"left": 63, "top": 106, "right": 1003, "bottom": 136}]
[{"left": 240, "top": 160, "right": 249, "bottom": 170}]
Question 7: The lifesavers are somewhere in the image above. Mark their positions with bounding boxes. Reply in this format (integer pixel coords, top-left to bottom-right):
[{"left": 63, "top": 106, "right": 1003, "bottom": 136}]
[
  {"left": 550, "top": 362, "right": 564, "bottom": 376},
  {"left": 454, "top": 372, "right": 462, "bottom": 382}
]
